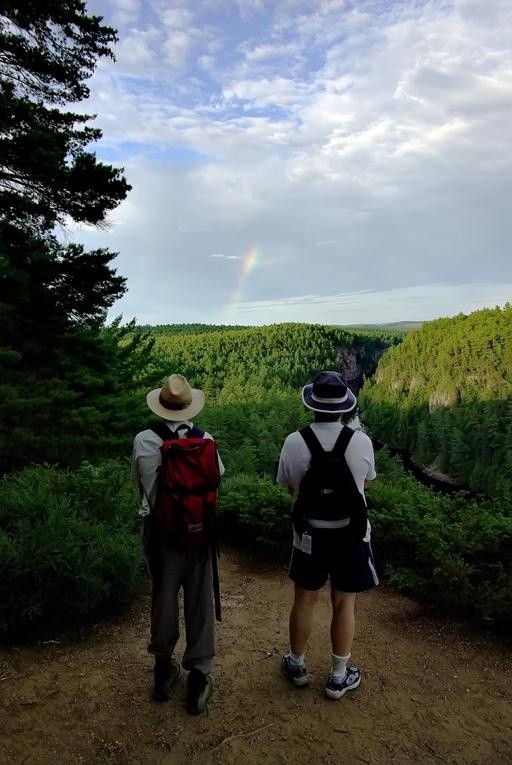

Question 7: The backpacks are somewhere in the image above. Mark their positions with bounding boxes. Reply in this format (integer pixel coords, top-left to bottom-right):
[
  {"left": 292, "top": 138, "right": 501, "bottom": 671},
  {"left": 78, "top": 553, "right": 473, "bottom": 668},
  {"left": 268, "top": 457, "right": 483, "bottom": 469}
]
[
  {"left": 142, "top": 423, "right": 219, "bottom": 553},
  {"left": 292, "top": 425, "right": 368, "bottom": 552}
]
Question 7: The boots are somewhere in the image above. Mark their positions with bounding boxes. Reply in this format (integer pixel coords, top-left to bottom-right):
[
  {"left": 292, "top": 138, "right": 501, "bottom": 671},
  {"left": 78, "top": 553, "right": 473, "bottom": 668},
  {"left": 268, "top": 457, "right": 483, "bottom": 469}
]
[
  {"left": 186, "top": 668, "right": 212, "bottom": 715},
  {"left": 151, "top": 662, "right": 181, "bottom": 701}
]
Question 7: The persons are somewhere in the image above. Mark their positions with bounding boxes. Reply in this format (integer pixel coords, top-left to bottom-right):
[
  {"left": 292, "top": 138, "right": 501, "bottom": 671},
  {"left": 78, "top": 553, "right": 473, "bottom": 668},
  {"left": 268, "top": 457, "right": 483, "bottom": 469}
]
[
  {"left": 129, "top": 373, "right": 224, "bottom": 715},
  {"left": 275, "top": 370, "right": 377, "bottom": 700}
]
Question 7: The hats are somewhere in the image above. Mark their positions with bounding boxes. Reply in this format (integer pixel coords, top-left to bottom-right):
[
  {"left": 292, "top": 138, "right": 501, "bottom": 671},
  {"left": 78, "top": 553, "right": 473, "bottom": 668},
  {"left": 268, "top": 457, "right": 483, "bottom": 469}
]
[
  {"left": 301, "top": 370, "right": 357, "bottom": 414},
  {"left": 146, "top": 374, "right": 205, "bottom": 422}
]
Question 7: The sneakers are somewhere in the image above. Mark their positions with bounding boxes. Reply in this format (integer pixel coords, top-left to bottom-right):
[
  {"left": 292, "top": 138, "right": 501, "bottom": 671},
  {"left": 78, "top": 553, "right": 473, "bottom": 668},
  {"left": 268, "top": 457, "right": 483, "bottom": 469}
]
[
  {"left": 280, "top": 654, "right": 308, "bottom": 686},
  {"left": 324, "top": 667, "right": 362, "bottom": 699}
]
